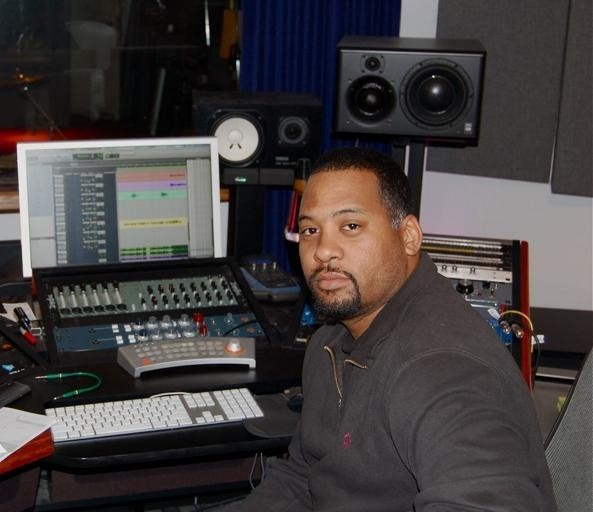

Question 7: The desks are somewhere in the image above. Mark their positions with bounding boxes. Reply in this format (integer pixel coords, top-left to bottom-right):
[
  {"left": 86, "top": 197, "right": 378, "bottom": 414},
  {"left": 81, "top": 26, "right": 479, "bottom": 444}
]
[{"left": 1, "top": 240, "right": 530, "bottom": 511}]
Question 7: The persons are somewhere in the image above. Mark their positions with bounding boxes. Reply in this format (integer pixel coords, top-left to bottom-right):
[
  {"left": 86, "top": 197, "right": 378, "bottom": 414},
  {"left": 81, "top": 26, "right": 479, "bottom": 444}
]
[{"left": 219, "top": 147, "right": 559, "bottom": 512}]
